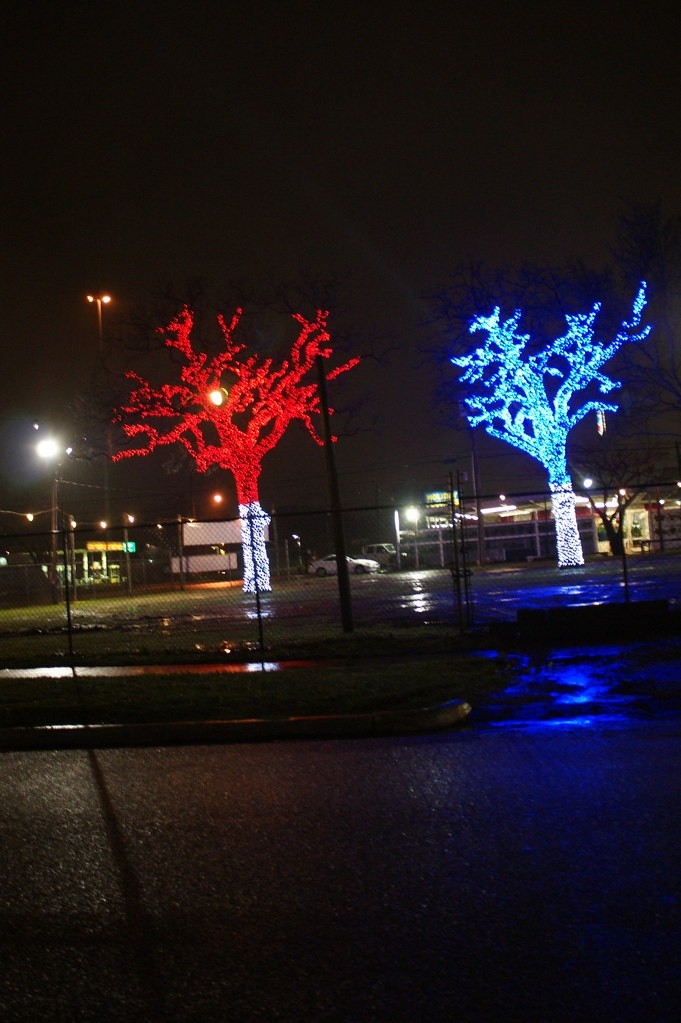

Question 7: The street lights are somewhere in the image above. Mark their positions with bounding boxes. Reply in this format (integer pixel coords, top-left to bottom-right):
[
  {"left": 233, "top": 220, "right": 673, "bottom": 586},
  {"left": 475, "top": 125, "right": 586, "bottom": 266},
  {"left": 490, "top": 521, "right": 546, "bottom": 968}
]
[{"left": 35, "top": 436, "right": 64, "bottom": 608}]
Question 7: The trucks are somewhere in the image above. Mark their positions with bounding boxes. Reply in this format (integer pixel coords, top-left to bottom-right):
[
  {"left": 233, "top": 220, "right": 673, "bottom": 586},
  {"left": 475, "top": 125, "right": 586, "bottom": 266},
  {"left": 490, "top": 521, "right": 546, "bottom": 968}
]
[{"left": 354, "top": 544, "right": 409, "bottom": 569}]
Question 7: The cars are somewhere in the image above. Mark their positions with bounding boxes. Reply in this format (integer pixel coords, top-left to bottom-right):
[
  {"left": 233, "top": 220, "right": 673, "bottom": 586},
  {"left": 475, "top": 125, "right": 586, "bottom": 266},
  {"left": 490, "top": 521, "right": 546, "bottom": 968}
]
[{"left": 307, "top": 553, "right": 379, "bottom": 580}]
